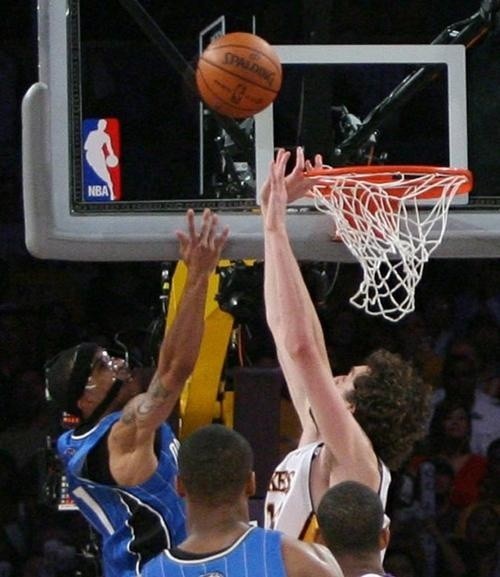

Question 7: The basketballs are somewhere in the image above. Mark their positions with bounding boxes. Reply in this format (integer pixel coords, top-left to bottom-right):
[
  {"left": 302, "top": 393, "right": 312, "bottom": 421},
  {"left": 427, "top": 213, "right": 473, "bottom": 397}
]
[{"left": 196, "top": 33, "right": 282, "bottom": 118}]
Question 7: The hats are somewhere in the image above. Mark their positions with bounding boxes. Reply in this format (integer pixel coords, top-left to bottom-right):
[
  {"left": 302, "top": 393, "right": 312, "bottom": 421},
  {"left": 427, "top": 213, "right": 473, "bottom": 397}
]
[{"left": 44, "top": 340, "right": 97, "bottom": 412}]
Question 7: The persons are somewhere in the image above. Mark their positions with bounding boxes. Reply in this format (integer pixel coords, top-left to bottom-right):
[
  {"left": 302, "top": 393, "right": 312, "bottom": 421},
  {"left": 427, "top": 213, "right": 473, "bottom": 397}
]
[{"left": 1, "top": 138, "right": 499, "bottom": 576}]
